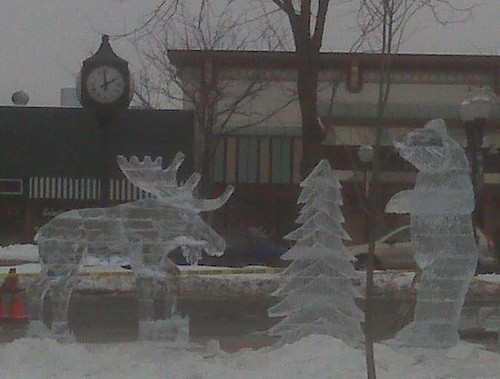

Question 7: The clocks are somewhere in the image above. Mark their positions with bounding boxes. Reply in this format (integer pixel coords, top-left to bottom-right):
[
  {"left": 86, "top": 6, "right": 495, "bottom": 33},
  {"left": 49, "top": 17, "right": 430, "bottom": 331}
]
[{"left": 86, "top": 67, "right": 125, "bottom": 104}]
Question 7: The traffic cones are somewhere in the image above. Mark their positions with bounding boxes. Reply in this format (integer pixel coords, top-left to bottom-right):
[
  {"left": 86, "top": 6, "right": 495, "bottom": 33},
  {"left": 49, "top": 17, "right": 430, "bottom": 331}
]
[{"left": 1, "top": 268, "right": 31, "bottom": 320}]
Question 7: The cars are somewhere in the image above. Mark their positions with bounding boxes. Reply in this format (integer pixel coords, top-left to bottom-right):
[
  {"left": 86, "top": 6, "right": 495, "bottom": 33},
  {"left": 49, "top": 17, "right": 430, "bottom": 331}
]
[{"left": 343, "top": 222, "right": 497, "bottom": 276}]
